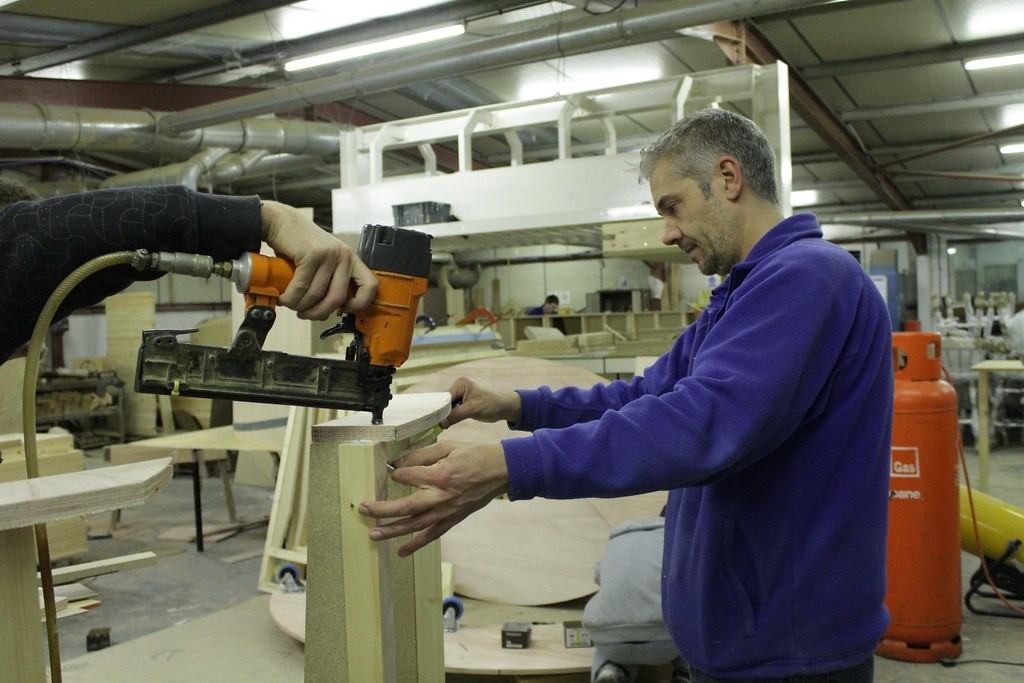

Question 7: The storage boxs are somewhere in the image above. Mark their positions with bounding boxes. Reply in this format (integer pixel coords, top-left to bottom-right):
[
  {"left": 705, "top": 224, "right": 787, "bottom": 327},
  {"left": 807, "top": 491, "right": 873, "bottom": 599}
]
[{"left": 391, "top": 202, "right": 451, "bottom": 227}]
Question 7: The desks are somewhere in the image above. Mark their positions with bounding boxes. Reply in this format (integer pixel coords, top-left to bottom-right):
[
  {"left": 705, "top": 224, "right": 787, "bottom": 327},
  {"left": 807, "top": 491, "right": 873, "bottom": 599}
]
[
  {"left": 128, "top": 425, "right": 285, "bottom": 553},
  {"left": 968, "top": 360, "right": 1024, "bottom": 495}
]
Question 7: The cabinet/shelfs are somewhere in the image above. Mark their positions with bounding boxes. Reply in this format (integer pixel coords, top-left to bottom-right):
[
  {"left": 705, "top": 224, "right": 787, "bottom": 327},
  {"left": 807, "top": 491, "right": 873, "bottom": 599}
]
[
  {"left": 35, "top": 375, "right": 125, "bottom": 450},
  {"left": 586, "top": 288, "right": 642, "bottom": 313}
]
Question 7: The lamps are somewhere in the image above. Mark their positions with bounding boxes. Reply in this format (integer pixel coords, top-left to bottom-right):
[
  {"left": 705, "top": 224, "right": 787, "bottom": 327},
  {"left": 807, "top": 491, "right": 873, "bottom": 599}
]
[
  {"left": 278, "top": 19, "right": 466, "bottom": 73},
  {"left": 962, "top": 50, "right": 1024, "bottom": 70}
]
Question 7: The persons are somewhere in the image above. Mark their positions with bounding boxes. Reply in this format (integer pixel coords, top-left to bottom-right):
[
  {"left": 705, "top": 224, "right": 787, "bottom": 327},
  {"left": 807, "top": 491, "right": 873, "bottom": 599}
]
[
  {"left": 529, "top": 294, "right": 559, "bottom": 314},
  {"left": 582, "top": 505, "right": 691, "bottom": 683},
  {"left": 358, "top": 112, "right": 894, "bottom": 683},
  {"left": 0, "top": 178, "right": 379, "bottom": 364}
]
